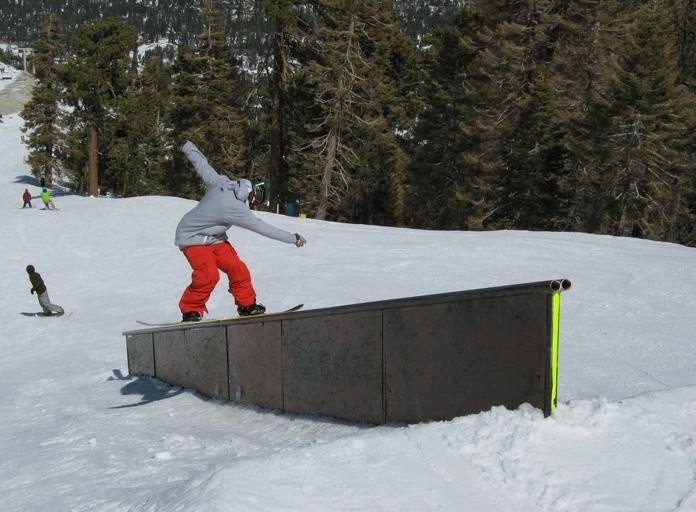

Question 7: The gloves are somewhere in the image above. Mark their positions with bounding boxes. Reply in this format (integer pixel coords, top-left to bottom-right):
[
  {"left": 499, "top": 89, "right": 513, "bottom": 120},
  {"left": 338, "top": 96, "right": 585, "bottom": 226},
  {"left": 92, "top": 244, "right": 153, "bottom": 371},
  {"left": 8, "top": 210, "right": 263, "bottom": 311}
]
[{"left": 294, "top": 233, "right": 307, "bottom": 248}]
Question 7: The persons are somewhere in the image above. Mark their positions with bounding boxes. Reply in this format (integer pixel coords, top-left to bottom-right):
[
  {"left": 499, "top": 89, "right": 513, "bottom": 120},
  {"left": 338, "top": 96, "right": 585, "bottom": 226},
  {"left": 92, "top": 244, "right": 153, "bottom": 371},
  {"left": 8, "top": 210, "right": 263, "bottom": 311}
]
[
  {"left": 22, "top": 188, "right": 33, "bottom": 209},
  {"left": 26, "top": 265, "right": 63, "bottom": 316},
  {"left": 172, "top": 137, "right": 308, "bottom": 323},
  {"left": 40, "top": 188, "right": 52, "bottom": 209}
]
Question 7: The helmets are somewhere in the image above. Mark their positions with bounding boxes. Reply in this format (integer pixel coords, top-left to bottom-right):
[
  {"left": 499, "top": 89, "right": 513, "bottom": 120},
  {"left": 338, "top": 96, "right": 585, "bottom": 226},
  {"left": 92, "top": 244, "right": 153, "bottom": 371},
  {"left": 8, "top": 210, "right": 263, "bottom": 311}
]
[{"left": 249, "top": 177, "right": 271, "bottom": 207}]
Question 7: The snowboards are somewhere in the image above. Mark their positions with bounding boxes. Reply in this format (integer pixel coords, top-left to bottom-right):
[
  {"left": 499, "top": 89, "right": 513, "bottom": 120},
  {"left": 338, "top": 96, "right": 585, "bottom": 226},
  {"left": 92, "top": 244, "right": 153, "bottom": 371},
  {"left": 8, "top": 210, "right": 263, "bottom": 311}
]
[
  {"left": 38, "top": 207, "right": 60, "bottom": 211},
  {"left": 134, "top": 304, "right": 303, "bottom": 326},
  {"left": 34, "top": 311, "right": 71, "bottom": 316}
]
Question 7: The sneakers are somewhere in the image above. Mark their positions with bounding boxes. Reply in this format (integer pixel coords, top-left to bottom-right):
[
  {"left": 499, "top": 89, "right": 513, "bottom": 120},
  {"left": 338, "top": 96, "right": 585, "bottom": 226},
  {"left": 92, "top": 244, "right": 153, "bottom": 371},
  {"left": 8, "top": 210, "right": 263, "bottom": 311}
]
[
  {"left": 236, "top": 303, "right": 265, "bottom": 315},
  {"left": 182, "top": 311, "right": 202, "bottom": 322}
]
